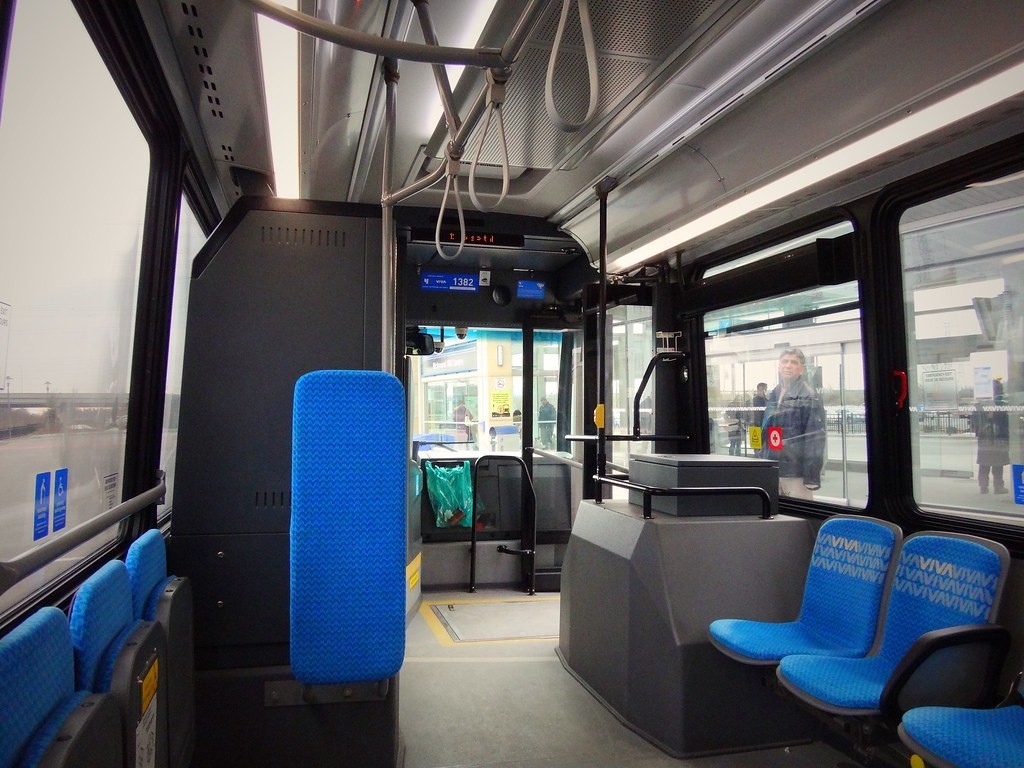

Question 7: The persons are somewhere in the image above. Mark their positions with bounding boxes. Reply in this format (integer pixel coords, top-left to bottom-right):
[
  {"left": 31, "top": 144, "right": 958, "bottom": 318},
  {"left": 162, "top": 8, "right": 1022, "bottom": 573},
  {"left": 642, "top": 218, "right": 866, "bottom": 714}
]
[
  {"left": 639, "top": 390, "right": 654, "bottom": 452},
  {"left": 538, "top": 397, "right": 555, "bottom": 449},
  {"left": 724, "top": 395, "right": 743, "bottom": 456},
  {"left": 736, "top": 382, "right": 769, "bottom": 456},
  {"left": 758, "top": 348, "right": 826, "bottom": 502},
  {"left": 464, "top": 414, "right": 473, "bottom": 440},
  {"left": 967, "top": 380, "right": 1011, "bottom": 495}
]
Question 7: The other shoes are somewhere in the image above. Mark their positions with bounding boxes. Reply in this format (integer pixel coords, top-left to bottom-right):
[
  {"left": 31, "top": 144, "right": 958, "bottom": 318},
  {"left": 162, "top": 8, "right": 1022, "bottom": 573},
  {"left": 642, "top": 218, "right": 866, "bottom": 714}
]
[
  {"left": 982, "top": 488, "right": 990, "bottom": 494},
  {"left": 994, "top": 487, "right": 1009, "bottom": 494}
]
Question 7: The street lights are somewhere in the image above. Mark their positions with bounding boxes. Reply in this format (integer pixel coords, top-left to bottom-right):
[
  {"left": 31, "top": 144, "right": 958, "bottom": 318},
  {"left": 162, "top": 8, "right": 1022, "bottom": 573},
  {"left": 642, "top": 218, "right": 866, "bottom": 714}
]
[
  {"left": 44, "top": 381, "right": 53, "bottom": 433},
  {"left": 6, "top": 376, "right": 14, "bottom": 439}
]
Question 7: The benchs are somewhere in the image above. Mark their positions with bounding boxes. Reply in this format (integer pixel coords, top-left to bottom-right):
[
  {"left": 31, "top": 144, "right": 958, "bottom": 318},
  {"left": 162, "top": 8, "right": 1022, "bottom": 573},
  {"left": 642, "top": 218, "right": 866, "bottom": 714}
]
[
  {"left": 703, "top": 514, "right": 1024, "bottom": 768},
  {"left": 0, "top": 526, "right": 207, "bottom": 768}
]
